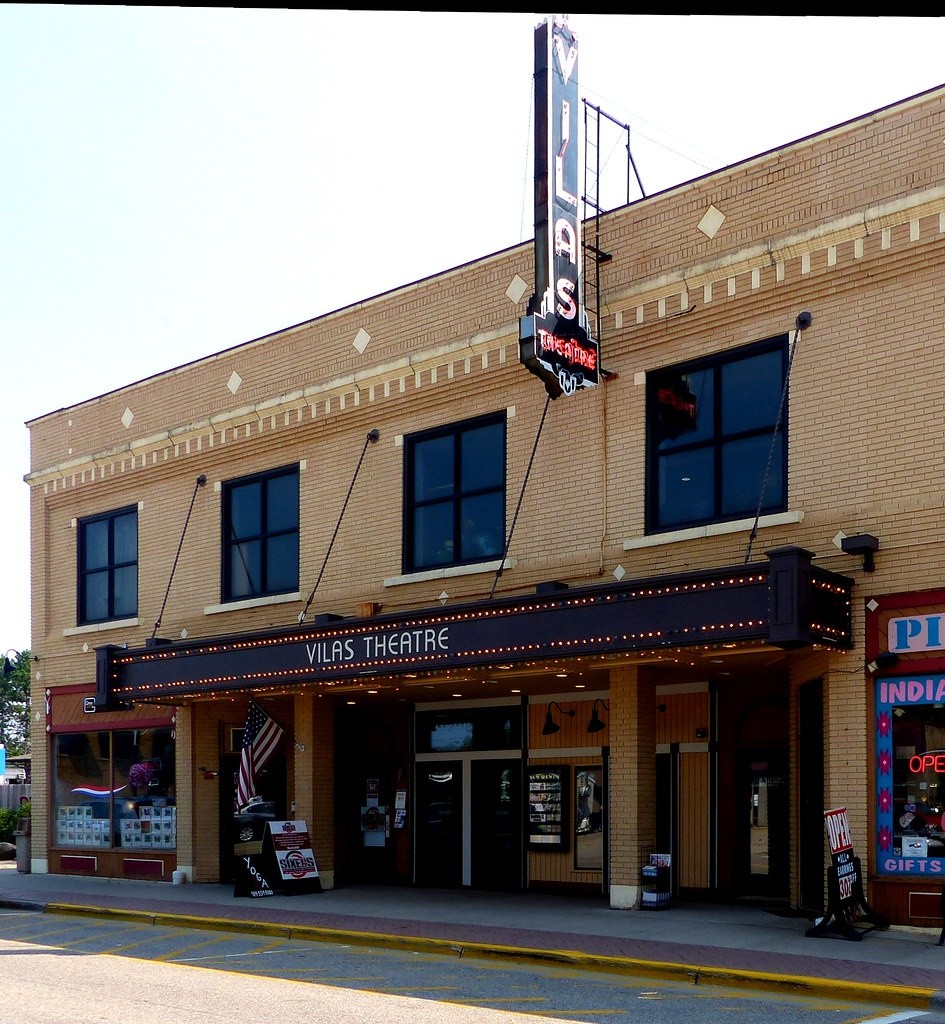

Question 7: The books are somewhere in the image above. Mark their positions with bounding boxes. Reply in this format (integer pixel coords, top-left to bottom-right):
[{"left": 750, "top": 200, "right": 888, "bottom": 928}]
[
  {"left": 642, "top": 854, "right": 672, "bottom": 876},
  {"left": 642, "top": 890, "right": 671, "bottom": 907}
]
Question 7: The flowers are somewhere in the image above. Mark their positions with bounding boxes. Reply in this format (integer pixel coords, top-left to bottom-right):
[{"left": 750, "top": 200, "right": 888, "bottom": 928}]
[{"left": 128, "top": 763, "right": 154, "bottom": 787}]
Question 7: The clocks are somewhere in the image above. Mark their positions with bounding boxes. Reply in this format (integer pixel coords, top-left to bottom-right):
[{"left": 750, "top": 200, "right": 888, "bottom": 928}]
[{"left": 238, "top": 827, "right": 254, "bottom": 841}]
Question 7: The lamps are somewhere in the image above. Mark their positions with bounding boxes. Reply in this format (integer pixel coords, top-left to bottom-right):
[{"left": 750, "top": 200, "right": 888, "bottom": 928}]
[
  {"left": 2, "top": 649, "right": 40, "bottom": 674},
  {"left": 587, "top": 698, "right": 610, "bottom": 733},
  {"left": 541, "top": 701, "right": 576, "bottom": 735}
]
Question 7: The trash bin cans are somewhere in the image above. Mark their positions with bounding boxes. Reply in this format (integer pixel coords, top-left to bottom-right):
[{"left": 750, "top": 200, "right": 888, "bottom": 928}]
[
  {"left": 12, "top": 817, "right": 31, "bottom": 873},
  {"left": 642, "top": 867, "right": 671, "bottom": 909}
]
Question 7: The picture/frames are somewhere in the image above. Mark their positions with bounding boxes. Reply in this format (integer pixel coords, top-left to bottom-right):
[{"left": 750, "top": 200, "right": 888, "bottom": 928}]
[{"left": 526, "top": 764, "right": 571, "bottom": 854}]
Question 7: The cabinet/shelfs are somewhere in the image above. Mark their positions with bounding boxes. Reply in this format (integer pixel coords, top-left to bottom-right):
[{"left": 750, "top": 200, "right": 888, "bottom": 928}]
[{"left": 641, "top": 845, "right": 671, "bottom": 912}]
